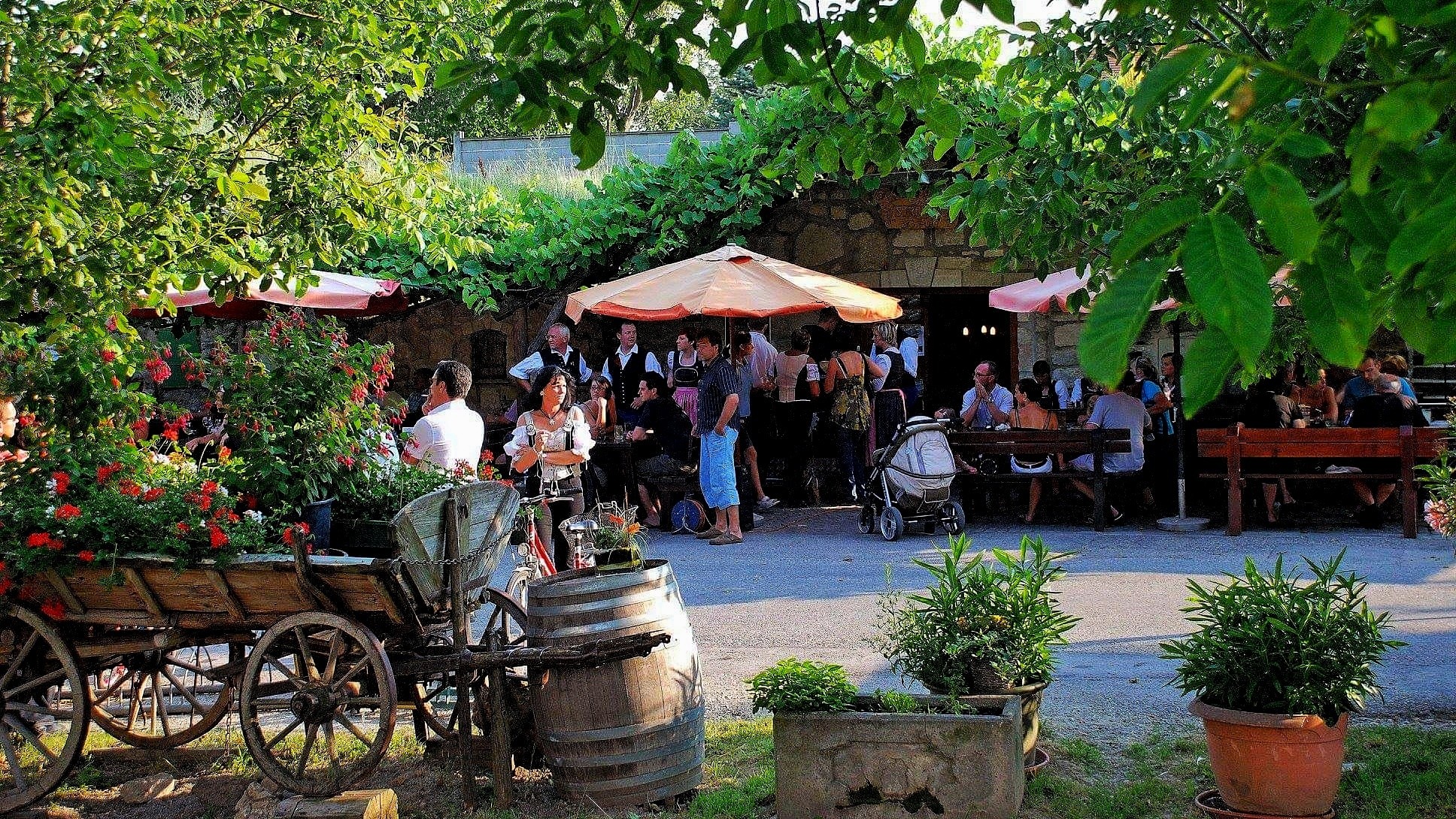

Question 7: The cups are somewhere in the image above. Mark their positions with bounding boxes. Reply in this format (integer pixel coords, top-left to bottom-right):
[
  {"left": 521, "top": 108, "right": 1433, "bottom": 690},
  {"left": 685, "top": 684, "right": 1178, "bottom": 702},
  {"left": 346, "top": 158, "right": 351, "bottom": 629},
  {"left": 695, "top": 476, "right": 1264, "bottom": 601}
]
[{"left": 614, "top": 425, "right": 623, "bottom": 441}]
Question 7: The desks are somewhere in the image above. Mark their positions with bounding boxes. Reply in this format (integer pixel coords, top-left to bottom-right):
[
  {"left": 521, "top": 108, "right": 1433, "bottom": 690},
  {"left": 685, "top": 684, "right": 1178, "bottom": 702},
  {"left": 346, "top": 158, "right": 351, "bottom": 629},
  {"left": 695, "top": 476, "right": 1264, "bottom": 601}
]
[{"left": 593, "top": 440, "right": 654, "bottom": 518}]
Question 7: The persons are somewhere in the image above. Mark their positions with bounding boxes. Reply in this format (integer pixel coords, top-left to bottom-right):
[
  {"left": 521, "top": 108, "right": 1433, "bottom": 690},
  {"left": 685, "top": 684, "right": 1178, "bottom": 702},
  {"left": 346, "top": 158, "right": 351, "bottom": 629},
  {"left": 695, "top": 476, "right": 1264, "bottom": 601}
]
[
  {"left": 627, "top": 371, "right": 702, "bottom": 529},
  {"left": 668, "top": 327, "right": 707, "bottom": 439},
  {"left": 958, "top": 358, "right": 1014, "bottom": 513},
  {"left": 1033, "top": 352, "right": 1187, "bottom": 519},
  {"left": 509, "top": 323, "right": 604, "bottom": 504},
  {"left": 184, "top": 384, "right": 233, "bottom": 454},
  {"left": 824, "top": 326, "right": 886, "bottom": 506},
  {"left": 1063, "top": 373, "right": 1153, "bottom": 530},
  {"left": 349, "top": 395, "right": 401, "bottom": 479},
  {"left": 580, "top": 376, "right": 619, "bottom": 512},
  {"left": 690, "top": 329, "right": 744, "bottom": 546},
  {"left": 404, "top": 358, "right": 486, "bottom": 483},
  {"left": 732, "top": 306, "right": 920, "bottom": 509},
  {"left": 600, "top": 319, "right": 665, "bottom": 433},
  {"left": 1230, "top": 345, "right": 1418, "bottom": 533},
  {"left": 502, "top": 365, "right": 596, "bottom": 568},
  {"left": 1008, "top": 378, "right": 1070, "bottom": 525}
]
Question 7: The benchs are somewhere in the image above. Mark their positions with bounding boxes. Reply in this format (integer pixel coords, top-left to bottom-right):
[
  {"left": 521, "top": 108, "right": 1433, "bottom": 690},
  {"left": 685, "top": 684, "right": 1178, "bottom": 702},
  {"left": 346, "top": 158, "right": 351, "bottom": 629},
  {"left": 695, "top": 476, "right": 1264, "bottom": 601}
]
[
  {"left": 1195, "top": 422, "right": 1449, "bottom": 537},
  {"left": 947, "top": 428, "right": 1132, "bottom": 531},
  {"left": 478, "top": 414, "right": 517, "bottom": 472},
  {"left": 658, "top": 477, "right": 701, "bottom": 532},
  {"left": 1411, "top": 367, "right": 1456, "bottom": 420}
]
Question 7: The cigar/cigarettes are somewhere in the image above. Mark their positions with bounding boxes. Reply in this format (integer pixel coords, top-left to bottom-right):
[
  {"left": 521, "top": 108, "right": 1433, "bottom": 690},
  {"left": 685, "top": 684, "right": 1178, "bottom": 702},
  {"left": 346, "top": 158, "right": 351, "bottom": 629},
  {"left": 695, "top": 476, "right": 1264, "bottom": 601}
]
[{"left": 420, "top": 390, "right": 432, "bottom": 397}]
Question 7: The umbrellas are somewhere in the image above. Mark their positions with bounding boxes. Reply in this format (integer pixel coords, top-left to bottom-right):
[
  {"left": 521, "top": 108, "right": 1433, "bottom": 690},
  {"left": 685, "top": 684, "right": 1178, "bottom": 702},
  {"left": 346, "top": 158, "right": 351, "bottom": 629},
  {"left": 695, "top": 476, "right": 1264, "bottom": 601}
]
[
  {"left": 569, "top": 243, "right": 906, "bottom": 370},
  {"left": 128, "top": 258, "right": 409, "bottom": 370},
  {"left": 1269, "top": 260, "right": 1302, "bottom": 307},
  {"left": 985, "top": 264, "right": 1193, "bottom": 315}
]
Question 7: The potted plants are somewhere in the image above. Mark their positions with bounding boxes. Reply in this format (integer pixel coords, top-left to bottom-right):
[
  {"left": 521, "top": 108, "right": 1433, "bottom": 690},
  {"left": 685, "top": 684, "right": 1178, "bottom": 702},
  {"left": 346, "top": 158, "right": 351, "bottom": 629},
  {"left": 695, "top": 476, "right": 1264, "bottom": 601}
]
[
  {"left": 1159, "top": 545, "right": 1411, "bottom": 818},
  {"left": 744, "top": 658, "right": 1026, "bottom": 819},
  {"left": 863, "top": 532, "right": 1083, "bottom": 768}
]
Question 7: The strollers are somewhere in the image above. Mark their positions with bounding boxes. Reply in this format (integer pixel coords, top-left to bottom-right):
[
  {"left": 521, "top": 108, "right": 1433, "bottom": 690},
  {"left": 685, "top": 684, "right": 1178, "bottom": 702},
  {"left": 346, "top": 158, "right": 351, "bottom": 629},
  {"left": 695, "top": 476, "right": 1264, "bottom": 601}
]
[{"left": 856, "top": 418, "right": 965, "bottom": 542}]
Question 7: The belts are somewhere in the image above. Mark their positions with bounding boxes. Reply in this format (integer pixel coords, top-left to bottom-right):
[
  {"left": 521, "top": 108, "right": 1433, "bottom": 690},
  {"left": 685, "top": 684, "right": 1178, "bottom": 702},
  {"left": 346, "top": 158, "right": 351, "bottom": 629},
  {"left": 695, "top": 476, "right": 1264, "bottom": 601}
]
[{"left": 1013, "top": 456, "right": 1048, "bottom": 469}]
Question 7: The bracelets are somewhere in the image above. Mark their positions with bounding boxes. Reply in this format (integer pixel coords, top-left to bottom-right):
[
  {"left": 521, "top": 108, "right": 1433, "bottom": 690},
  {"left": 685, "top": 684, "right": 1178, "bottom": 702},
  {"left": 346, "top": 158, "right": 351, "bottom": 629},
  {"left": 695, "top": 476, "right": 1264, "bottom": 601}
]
[{"left": 532, "top": 443, "right": 543, "bottom": 456}]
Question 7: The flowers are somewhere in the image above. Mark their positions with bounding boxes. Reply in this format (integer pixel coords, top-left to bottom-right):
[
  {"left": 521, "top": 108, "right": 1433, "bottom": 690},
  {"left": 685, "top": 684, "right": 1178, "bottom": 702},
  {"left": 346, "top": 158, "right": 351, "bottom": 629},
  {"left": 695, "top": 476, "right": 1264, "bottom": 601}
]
[
  {"left": 592, "top": 514, "right": 641, "bottom": 550},
  {"left": 0, "top": 307, "right": 553, "bottom": 618}
]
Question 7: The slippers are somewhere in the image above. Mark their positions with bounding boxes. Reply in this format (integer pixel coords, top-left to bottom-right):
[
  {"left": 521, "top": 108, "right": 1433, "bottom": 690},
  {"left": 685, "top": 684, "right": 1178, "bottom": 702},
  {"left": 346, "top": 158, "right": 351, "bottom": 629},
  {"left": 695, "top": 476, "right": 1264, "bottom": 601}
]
[
  {"left": 709, "top": 531, "right": 744, "bottom": 545},
  {"left": 642, "top": 520, "right": 659, "bottom": 529},
  {"left": 696, "top": 525, "right": 723, "bottom": 539}
]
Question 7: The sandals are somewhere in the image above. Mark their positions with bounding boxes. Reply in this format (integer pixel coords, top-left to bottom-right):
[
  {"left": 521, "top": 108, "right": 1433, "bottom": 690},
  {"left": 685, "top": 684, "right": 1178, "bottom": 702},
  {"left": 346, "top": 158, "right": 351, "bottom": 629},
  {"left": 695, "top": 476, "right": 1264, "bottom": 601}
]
[{"left": 757, "top": 496, "right": 780, "bottom": 510}]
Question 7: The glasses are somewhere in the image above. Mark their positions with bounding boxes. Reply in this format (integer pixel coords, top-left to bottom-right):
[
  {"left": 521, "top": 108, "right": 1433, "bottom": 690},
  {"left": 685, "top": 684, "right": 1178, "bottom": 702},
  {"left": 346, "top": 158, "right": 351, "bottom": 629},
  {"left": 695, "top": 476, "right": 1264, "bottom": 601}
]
[
  {"left": 972, "top": 373, "right": 989, "bottom": 378},
  {"left": 545, "top": 335, "right": 556, "bottom": 340},
  {"left": 428, "top": 378, "right": 440, "bottom": 384}
]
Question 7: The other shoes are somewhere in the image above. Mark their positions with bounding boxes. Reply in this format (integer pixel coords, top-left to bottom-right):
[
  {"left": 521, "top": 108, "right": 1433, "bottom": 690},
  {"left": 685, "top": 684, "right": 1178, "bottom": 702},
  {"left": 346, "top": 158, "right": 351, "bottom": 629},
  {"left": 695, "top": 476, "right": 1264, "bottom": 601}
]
[
  {"left": 1018, "top": 512, "right": 1033, "bottom": 525},
  {"left": 1081, "top": 513, "right": 1095, "bottom": 524},
  {"left": 1112, "top": 512, "right": 1123, "bottom": 522}
]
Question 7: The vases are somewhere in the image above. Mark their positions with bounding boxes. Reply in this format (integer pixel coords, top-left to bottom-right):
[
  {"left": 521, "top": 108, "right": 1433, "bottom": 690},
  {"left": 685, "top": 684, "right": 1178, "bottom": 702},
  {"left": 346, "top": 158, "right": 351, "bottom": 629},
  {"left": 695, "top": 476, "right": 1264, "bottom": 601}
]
[
  {"left": 331, "top": 518, "right": 394, "bottom": 548},
  {"left": 595, "top": 547, "right": 642, "bottom": 575},
  {"left": 296, "top": 495, "right": 336, "bottom": 551}
]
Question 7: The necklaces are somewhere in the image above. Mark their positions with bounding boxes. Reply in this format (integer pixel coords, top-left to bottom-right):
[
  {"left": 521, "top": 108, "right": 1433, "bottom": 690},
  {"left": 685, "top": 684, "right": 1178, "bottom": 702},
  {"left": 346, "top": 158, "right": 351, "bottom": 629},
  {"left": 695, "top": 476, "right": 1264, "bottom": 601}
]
[{"left": 539, "top": 407, "right": 562, "bottom": 426}]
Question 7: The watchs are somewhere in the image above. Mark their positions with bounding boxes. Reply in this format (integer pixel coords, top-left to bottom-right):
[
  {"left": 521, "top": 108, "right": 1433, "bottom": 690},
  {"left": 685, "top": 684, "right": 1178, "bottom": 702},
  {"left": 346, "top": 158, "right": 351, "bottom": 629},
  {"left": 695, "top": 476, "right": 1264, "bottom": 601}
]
[
  {"left": 983, "top": 397, "right": 996, "bottom": 404},
  {"left": 542, "top": 452, "right": 548, "bottom": 463}
]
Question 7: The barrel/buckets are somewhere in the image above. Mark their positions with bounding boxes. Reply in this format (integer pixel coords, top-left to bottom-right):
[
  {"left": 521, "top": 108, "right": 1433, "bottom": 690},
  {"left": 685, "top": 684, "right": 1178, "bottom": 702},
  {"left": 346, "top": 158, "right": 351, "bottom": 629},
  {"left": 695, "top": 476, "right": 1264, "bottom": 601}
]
[
  {"left": 671, "top": 500, "right": 708, "bottom": 534},
  {"left": 528, "top": 558, "right": 705, "bottom": 805}
]
